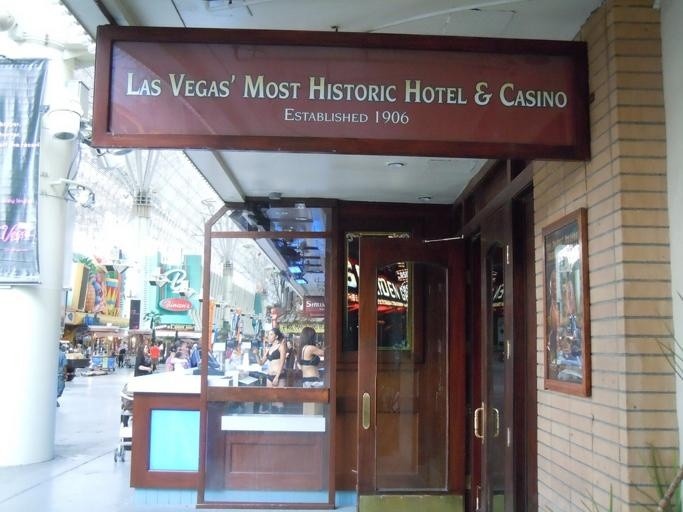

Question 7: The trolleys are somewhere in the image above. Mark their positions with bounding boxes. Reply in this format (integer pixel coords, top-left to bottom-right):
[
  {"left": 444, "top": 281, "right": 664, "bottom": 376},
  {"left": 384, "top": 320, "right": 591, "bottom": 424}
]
[{"left": 114, "top": 392, "right": 134, "bottom": 462}]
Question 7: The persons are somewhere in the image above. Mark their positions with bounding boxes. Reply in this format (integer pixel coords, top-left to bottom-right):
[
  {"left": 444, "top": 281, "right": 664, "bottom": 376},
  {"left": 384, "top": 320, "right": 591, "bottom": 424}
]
[
  {"left": 57, "top": 344, "right": 68, "bottom": 407},
  {"left": 64, "top": 341, "right": 92, "bottom": 358},
  {"left": 113, "top": 327, "right": 323, "bottom": 414}
]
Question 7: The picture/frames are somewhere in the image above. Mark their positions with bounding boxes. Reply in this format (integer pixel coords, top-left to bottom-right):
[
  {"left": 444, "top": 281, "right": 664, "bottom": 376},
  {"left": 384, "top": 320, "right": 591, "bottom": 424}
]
[{"left": 541, "top": 207, "right": 592, "bottom": 398}]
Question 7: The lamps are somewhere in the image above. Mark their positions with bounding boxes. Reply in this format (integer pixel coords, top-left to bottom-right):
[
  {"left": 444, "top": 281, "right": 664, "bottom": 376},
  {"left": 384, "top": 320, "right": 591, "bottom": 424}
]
[
  {"left": 277, "top": 246, "right": 324, "bottom": 285},
  {"left": 288, "top": 200, "right": 313, "bottom": 222}
]
